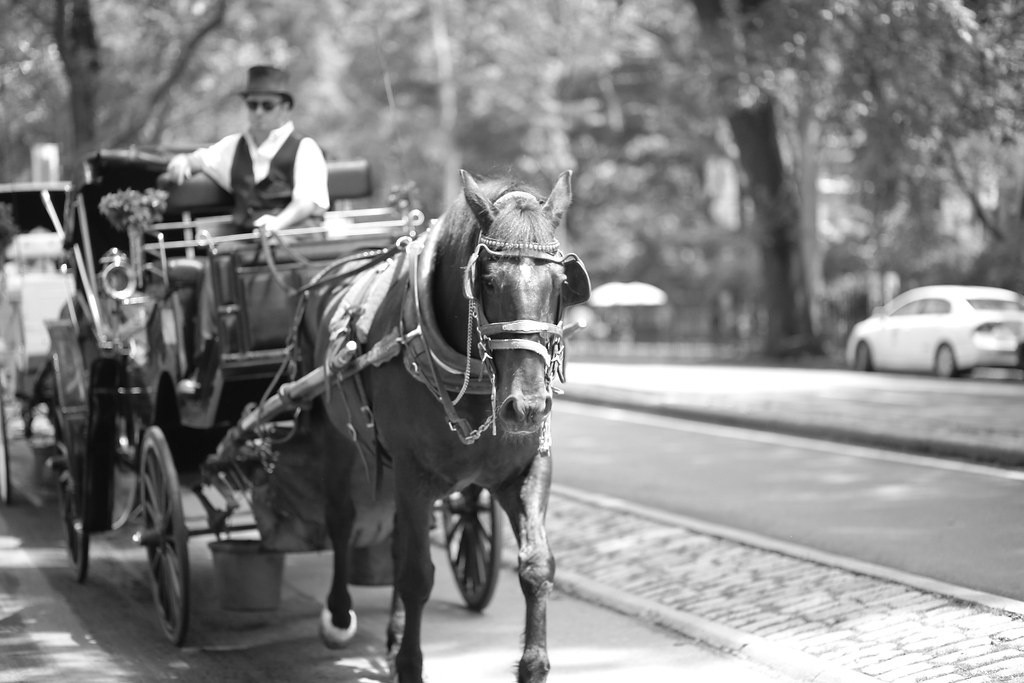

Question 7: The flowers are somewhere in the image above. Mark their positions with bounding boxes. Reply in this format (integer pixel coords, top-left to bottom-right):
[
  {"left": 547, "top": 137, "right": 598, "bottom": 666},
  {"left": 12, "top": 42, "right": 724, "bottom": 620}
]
[{"left": 99, "top": 185, "right": 168, "bottom": 230}]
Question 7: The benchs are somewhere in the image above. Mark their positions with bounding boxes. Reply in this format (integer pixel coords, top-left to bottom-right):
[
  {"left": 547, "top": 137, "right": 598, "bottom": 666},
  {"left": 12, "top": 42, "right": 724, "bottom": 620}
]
[{"left": 145, "top": 157, "right": 390, "bottom": 282}]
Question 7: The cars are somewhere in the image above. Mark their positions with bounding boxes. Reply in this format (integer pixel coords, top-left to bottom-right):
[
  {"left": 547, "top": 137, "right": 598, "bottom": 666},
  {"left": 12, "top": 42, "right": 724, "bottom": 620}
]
[{"left": 845, "top": 285, "right": 1024, "bottom": 379}]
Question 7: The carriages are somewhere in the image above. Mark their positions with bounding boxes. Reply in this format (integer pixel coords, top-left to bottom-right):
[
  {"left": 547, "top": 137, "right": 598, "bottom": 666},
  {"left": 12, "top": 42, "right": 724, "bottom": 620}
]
[{"left": 0, "top": 145, "right": 592, "bottom": 683}]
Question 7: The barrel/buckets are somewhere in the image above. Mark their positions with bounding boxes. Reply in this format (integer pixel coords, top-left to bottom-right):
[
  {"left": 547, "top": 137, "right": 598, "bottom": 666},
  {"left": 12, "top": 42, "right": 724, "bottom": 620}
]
[
  {"left": 347, "top": 530, "right": 397, "bottom": 586},
  {"left": 207, "top": 527, "right": 283, "bottom": 613}
]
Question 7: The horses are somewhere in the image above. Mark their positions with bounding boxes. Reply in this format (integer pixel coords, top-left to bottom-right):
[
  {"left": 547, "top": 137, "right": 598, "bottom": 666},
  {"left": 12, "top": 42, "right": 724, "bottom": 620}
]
[{"left": 299, "top": 165, "right": 593, "bottom": 683}]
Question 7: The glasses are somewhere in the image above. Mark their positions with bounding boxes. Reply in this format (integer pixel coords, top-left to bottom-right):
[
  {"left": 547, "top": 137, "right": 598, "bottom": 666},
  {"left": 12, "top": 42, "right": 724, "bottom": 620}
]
[{"left": 246, "top": 101, "right": 288, "bottom": 110}]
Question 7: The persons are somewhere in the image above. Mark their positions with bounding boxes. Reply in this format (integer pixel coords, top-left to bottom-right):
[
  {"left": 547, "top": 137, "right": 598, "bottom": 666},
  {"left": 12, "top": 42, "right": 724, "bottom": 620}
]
[{"left": 167, "top": 65, "right": 330, "bottom": 236}]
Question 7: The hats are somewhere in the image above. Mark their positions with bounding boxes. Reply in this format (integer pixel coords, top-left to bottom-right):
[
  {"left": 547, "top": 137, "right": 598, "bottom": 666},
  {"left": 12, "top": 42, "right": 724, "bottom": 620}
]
[{"left": 235, "top": 65, "right": 291, "bottom": 108}]
